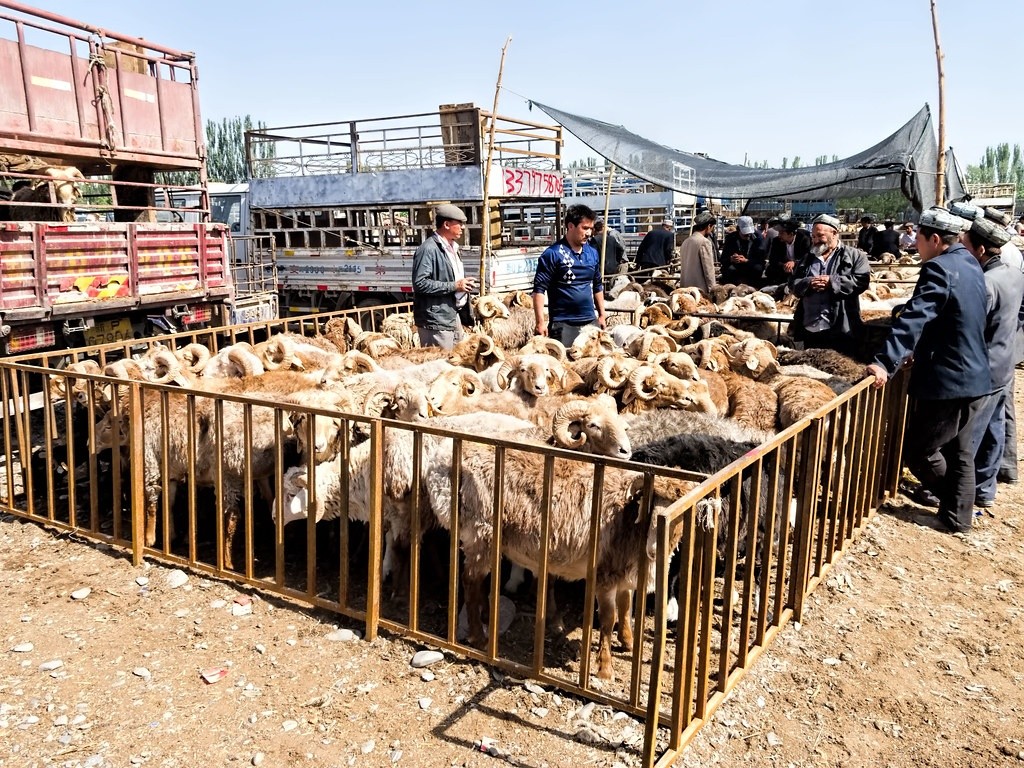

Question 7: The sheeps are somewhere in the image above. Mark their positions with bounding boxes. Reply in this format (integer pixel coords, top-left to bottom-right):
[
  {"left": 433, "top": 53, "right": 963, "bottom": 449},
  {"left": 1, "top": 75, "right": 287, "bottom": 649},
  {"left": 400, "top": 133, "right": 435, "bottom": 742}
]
[
  {"left": 51, "top": 262, "right": 868, "bottom": 685},
  {"left": 858, "top": 253, "right": 924, "bottom": 322}
]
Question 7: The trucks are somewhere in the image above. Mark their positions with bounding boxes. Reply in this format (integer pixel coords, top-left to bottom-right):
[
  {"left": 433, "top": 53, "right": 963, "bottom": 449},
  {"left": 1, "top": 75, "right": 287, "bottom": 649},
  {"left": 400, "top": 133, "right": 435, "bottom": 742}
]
[
  {"left": 154, "top": 106, "right": 738, "bottom": 346},
  {"left": 0, "top": 1, "right": 239, "bottom": 420}
]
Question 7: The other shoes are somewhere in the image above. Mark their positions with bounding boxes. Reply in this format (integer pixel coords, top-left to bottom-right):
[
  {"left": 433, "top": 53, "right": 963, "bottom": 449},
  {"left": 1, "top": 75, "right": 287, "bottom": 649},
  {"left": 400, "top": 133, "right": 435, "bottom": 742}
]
[
  {"left": 976, "top": 500, "right": 994, "bottom": 507},
  {"left": 918, "top": 487, "right": 940, "bottom": 508},
  {"left": 998, "top": 468, "right": 1018, "bottom": 486},
  {"left": 913, "top": 513, "right": 950, "bottom": 529}
]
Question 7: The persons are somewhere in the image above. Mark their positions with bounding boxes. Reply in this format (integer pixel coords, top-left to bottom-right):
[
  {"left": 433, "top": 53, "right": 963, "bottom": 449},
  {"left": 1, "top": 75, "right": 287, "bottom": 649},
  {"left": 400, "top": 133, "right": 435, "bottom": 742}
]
[
  {"left": 533, "top": 204, "right": 606, "bottom": 348},
  {"left": 863, "top": 209, "right": 992, "bottom": 533},
  {"left": 787, "top": 213, "right": 870, "bottom": 351},
  {"left": 720, "top": 215, "right": 766, "bottom": 287},
  {"left": 696, "top": 207, "right": 721, "bottom": 261},
  {"left": 765, "top": 220, "right": 812, "bottom": 286},
  {"left": 950, "top": 202, "right": 1024, "bottom": 506},
  {"left": 635, "top": 219, "right": 674, "bottom": 269},
  {"left": 589, "top": 216, "right": 630, "bottom": 273},
  {"left": 412, "top": 204, "right": 478, "bottom": 349},
  {"left": 753, "top": 218, "right": 779, "bottom": 238},
  {"left": 857, "top": 217, "right": 877, "bottom": 253},
  {"left": 680, "top": 212, "right": 717, "bottom": 286},
  {"left": 870, "top": 221, "right": 901, "bottom": 259},
  {"left": 898, "top": 222, "right": 917, "bottom": 246}
]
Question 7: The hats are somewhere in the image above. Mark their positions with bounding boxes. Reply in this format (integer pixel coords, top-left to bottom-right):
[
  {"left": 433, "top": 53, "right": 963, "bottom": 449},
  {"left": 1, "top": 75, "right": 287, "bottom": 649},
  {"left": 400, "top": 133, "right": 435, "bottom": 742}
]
[
  {"left": 918, "top": 209, "right": 963, "bottom": 234},
  {"left": 778, "top": 213, "right": 789, "bottom": 221},
  {"left": 986, "top": 207, "right": 1011, "bottom": 226},
  {"left": 436, "top": 204, "right": 467, "bottom": 221},
  {"left": 935, "top": 208, "right": 973, "bottom": 234},
  {"left": 972, "top": 217, "right": 1011, "bottom": 246},
  {"left": 694, "top": 213, "right": 712, "bottom": 224},
  {"left": 737, "top": 216, "right": 755, "bottom": 235},
  {"left": 904, "top": 222, "right": 914, "bottom": 227},
  {"left": 798, "top": 221, "right": 807, "bottom": 230},
  {"left": 813, "top": 214, "right": 840, "bottom": 230},
  {"left": 662, "top": 220, "right": 673, "bottom": 226},
  {"left": 951, "top": 201, "right": 984, "bottom": 220}
]
